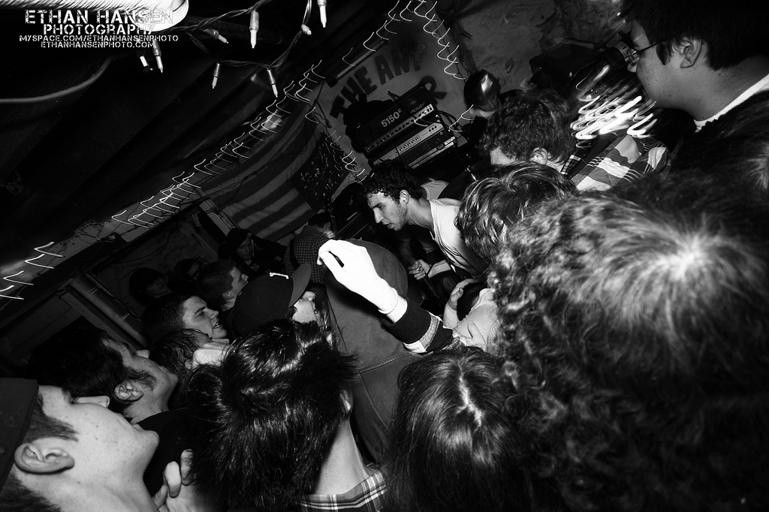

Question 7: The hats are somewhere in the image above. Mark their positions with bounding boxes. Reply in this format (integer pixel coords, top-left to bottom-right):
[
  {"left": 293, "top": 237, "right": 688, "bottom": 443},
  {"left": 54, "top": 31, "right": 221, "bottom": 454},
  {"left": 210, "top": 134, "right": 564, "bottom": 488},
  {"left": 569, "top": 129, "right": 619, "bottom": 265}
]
[
  {"left": 0, "top": 377, "right": 38, "bottom": 489},
  {"left": 234, "top": 262, "right": 312, "bottom": 324}
]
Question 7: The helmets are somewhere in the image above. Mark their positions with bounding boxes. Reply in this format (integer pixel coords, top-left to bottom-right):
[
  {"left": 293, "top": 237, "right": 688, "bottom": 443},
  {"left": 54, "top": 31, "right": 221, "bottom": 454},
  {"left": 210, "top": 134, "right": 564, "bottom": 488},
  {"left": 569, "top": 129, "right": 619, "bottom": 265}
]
[{"left": 465, "top": 69, "right": 500, "bottom": 110}]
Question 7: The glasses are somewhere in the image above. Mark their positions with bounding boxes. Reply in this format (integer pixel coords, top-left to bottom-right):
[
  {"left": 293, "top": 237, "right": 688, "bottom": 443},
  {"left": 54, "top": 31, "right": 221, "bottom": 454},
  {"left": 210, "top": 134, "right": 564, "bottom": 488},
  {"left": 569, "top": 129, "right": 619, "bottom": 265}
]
[{"left": 624, "top": 42, "right": 658, "bottom": 64}]
